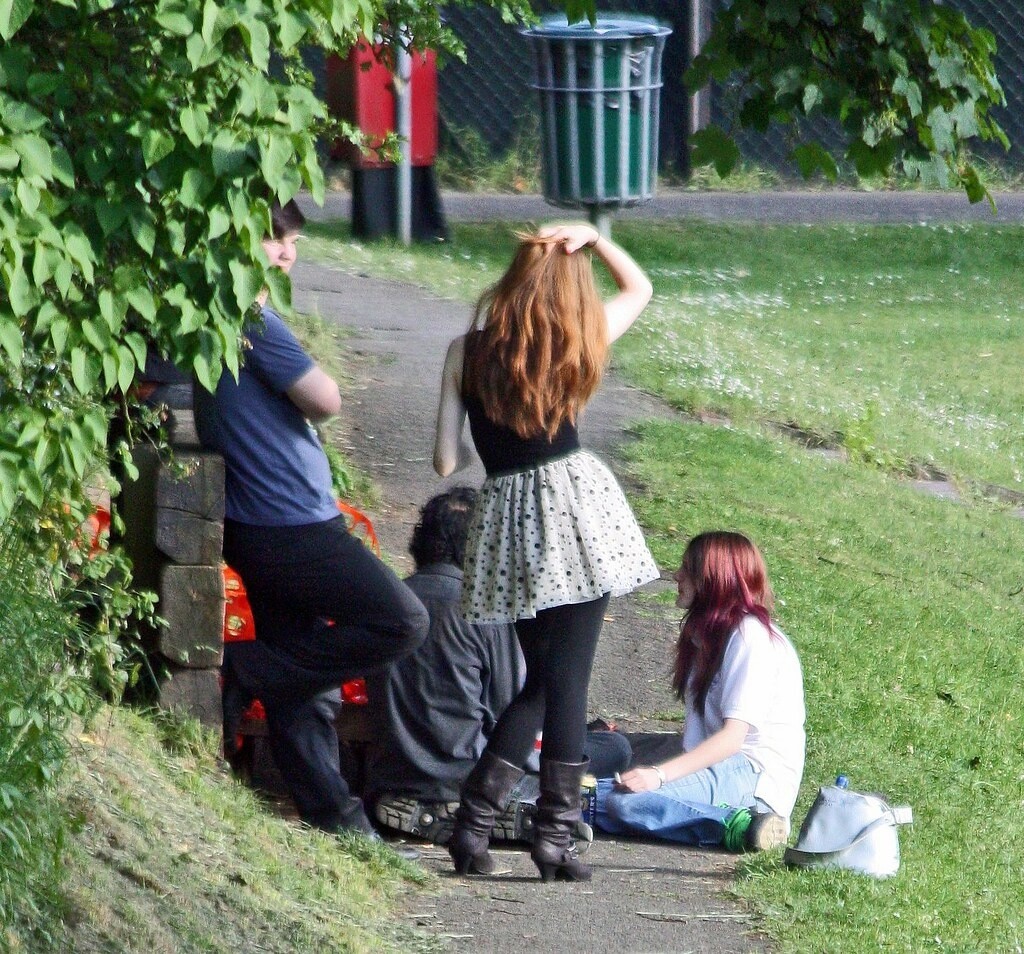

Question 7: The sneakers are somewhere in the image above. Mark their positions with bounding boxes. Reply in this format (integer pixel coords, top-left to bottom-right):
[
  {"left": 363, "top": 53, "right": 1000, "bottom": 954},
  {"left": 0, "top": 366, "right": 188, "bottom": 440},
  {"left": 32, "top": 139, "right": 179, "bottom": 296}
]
[{"left": 715, "top": 803, "right": 790, "bottom": 853}]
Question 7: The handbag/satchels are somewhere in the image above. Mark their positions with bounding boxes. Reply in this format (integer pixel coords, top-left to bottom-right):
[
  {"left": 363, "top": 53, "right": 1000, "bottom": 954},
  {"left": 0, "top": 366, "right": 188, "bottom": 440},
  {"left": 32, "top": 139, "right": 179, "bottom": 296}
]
[{"left": 784, "top": 784, "right": 912, "bottom": 881}]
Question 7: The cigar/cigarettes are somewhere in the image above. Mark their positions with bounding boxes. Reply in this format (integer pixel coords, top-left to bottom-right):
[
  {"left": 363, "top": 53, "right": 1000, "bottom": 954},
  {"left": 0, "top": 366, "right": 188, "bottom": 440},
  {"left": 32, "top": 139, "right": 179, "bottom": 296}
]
[{"left": 614, "top": 772, "right": 622, "bottom": 784}]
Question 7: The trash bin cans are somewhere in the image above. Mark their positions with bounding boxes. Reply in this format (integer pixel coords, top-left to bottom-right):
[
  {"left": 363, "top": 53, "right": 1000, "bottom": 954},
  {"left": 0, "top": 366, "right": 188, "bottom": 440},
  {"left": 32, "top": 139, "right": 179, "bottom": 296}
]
[{"left": 517, "top": 17, "right": 674, "bottom": 212}]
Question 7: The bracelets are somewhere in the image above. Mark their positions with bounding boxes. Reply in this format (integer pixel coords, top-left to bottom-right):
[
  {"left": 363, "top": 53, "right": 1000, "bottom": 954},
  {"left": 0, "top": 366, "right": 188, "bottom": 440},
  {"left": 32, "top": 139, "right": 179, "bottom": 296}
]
[
  {"left": 587, "top": 232, "right": 600, "bottom": 249},
  {"left": 651, "top": 764, "right": 665, "bottom": 788}
]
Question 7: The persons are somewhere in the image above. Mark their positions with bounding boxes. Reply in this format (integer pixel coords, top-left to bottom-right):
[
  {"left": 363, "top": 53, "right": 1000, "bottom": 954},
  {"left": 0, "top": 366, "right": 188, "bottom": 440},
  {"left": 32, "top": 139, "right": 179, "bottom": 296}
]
[
  {"left": 433, "top": 219, "right": 660, "bottom": 882},
  {"left": 192, "top": 193, "right": 431, "bottom": 860},
  {"left": 584, "top": 531, "right": 806, "bottom": 852},
  {"left": 360, "top": 486, "right": 631, "bottom": 857}
]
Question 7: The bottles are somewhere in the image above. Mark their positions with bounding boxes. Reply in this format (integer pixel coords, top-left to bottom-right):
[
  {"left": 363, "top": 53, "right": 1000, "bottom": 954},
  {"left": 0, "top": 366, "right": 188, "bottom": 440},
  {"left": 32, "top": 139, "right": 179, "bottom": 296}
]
[{"left": 836, "top": 775, "right": 849, "bottom": 790}]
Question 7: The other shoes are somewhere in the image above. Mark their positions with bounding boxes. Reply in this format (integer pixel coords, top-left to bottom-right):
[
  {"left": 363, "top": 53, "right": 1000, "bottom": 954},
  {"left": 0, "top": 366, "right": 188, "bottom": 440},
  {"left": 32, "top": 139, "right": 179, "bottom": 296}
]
[
  {"left": 488, "top": 800, "right": 594, "bottom": 855},
  {"left": 374, "top": 791, "right": 459, "bottom": 845},
  {"left": 221, "top": 640, "right": 255, "bottom": 753},
  {"left": 365, "top": 827, "right": 421, "bottom": 862}
]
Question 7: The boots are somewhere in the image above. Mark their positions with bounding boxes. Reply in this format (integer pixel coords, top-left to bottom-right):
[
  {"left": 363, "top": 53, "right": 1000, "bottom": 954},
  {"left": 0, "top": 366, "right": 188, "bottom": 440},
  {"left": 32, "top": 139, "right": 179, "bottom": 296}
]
[
  {"left": 448, "top": 749, "right": 527, "bottom": 874},
  {"left": 532, "top": 752, "right": 593, "bottom": 883}
]
